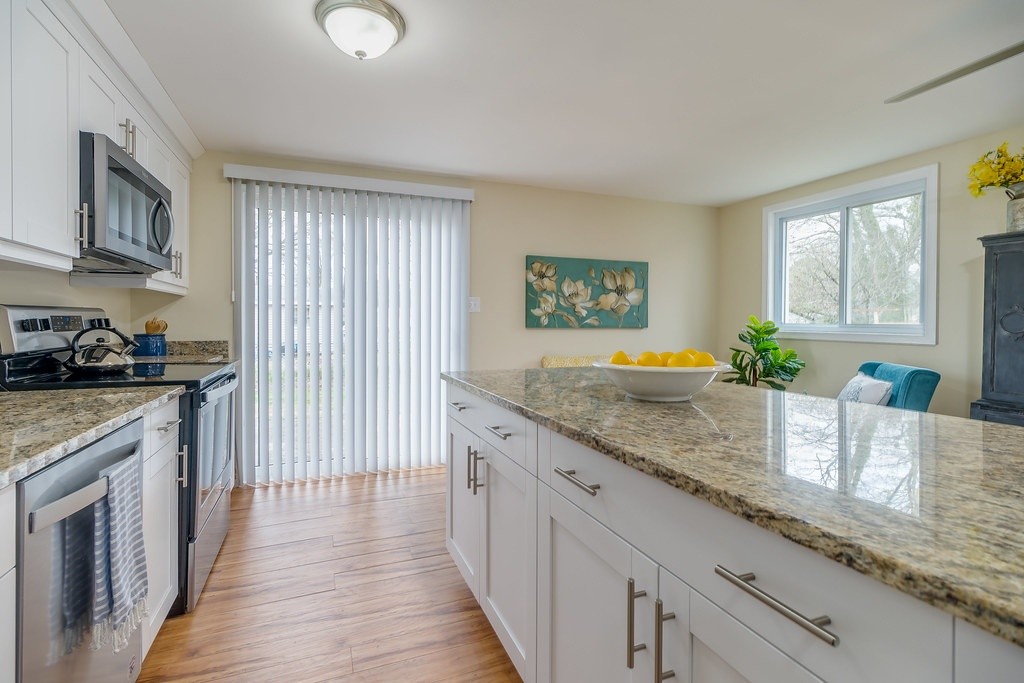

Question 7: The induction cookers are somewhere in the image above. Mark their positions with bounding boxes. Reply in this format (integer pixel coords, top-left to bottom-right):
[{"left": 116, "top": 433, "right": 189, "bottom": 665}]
[{"left": 0, "top": 305, "right": 241, "bottom": 388}]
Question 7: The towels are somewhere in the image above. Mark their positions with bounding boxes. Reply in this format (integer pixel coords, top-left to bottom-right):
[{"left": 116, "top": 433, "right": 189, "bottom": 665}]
[
  {"left": 46, "top": 489, "right": 93, "bottom": 670},
  {"left": 90, "top": 448, "right": 150, "bottom": 657}
]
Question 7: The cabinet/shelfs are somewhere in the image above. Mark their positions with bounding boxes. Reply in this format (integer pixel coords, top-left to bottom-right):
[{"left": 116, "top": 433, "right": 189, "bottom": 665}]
[
  {"left": 0, "top": 0, "right": 205, "bottom": 298},
  {"left": 970, "top": 233, "right": 1024, "bottom": 427},
  {"left": 0, "top": 373, "right": 240, "bottom": 683},
  {"left": 440, "top": 381, "right": 1024, "bottom": 683}
]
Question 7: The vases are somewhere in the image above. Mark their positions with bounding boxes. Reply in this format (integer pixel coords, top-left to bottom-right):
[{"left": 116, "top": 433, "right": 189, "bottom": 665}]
[{"left": 1006, "top": 182, "right": 1024, "bottom": 233}]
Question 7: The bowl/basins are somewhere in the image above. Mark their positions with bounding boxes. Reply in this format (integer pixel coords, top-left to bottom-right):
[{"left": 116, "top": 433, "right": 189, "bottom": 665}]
[{"left": 593, "top": 360, "right": 733, "bottom": 402}]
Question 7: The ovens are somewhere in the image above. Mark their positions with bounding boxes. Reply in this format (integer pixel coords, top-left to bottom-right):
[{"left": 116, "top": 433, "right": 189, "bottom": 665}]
[
  {"left": 17, "top": 415, "right": 141, "bottom": 683},
  {"left": 170, "top": 370, "right": 239, "bottom": 617}
]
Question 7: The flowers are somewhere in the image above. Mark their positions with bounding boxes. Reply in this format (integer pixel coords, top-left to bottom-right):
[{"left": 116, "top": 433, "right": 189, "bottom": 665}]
[{"left": 967, "top": 140, "right": 1024, "bottom": 199}]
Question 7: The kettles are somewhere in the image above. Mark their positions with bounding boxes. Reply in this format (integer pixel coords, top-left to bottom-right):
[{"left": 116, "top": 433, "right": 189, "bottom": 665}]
[{"left": 62, "top": 318, "right": 140, "bottom": 375}]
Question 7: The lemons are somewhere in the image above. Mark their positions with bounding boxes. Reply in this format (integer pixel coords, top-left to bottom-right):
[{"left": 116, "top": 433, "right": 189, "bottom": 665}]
[{"left": 609, "top": 349, "right": 716, "bottom": 367}]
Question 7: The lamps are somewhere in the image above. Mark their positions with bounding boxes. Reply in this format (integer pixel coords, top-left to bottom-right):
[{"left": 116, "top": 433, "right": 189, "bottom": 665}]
[{"left": 315, "top": 1, "right": 406, "bottom": 59}]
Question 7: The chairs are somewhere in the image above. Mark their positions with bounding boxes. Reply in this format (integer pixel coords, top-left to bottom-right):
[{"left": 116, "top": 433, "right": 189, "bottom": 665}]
[{"left": 858, "top": 361, "right": 942, "bottom": 412}]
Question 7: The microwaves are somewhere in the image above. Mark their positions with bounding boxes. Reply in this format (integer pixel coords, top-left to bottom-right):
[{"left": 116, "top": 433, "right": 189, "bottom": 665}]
[{"left": 70, "top": 132, "right": 172, "bottom": 275}]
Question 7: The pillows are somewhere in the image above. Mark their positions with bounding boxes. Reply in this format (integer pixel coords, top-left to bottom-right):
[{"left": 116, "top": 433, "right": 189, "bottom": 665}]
[{"left": 835, "top": 371, "right": 893, "bottom": 405}]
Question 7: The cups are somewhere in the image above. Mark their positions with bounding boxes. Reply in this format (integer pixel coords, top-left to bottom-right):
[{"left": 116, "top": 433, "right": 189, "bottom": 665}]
[{"left": 132, "top": 333, "right": 167, "bottom": 356}]
